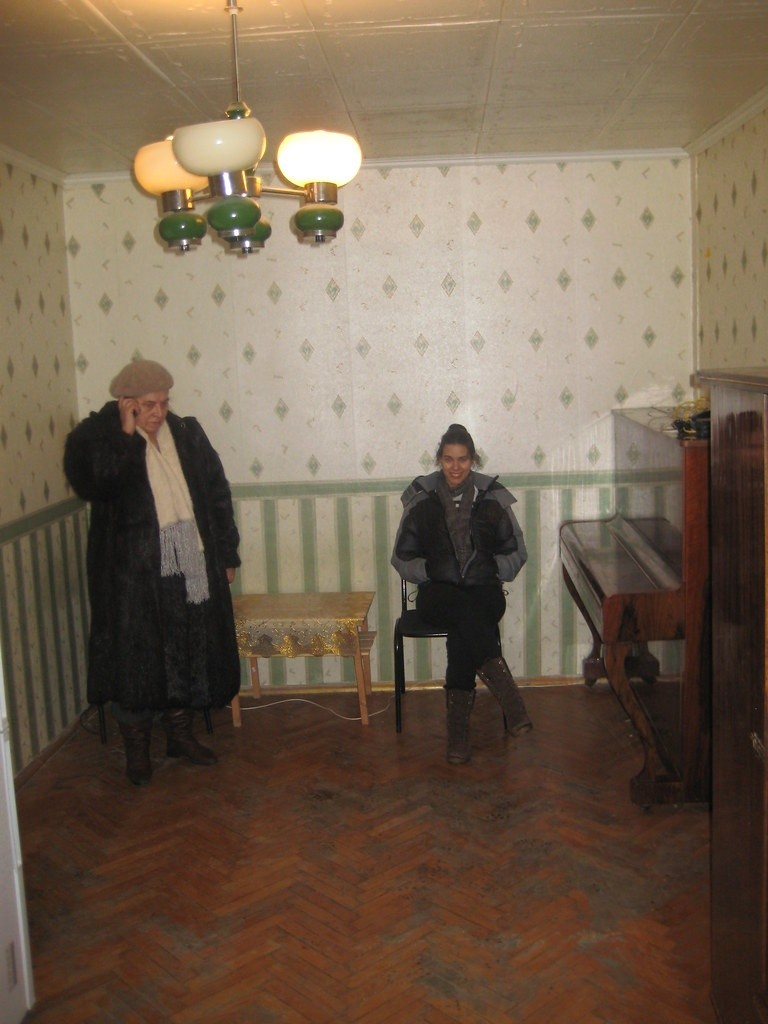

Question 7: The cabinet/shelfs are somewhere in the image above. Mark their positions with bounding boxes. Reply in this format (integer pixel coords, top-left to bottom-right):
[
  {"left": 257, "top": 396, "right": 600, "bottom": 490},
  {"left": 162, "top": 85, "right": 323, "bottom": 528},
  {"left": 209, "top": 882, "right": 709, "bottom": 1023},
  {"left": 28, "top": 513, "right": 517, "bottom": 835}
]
[{"left": 691, "top": 367, "right": 767, "bottom": 1024}]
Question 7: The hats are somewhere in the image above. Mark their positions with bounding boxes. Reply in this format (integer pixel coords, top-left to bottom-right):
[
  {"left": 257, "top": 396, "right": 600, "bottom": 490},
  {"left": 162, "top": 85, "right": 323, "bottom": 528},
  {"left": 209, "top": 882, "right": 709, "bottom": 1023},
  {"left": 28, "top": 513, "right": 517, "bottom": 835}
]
[{"left": 109, "top": 360, "right": 173, "bottom": 399}]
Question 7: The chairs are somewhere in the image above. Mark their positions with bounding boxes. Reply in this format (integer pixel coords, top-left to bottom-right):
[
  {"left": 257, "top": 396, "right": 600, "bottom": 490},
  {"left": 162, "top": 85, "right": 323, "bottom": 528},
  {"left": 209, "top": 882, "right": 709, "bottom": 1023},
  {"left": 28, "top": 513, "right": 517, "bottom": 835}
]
[{"left": 395, "top": 577, "right": 508, "bottom": 735}]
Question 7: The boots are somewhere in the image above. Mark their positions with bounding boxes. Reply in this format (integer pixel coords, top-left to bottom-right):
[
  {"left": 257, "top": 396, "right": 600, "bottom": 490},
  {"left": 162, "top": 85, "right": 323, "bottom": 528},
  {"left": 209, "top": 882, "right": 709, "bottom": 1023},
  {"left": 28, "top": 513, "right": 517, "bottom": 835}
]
[
  {"left": 445, "top": 689, "right": 474, "bottom": 764},
  {"left": 477, "top": 656, "right": 533, "bottom": 737},
  {"left": 164, "top": 709, "right": 218, "bottom": 765},
  {"left": 111, "top": 703, "right": 152, "bottom": 785}
]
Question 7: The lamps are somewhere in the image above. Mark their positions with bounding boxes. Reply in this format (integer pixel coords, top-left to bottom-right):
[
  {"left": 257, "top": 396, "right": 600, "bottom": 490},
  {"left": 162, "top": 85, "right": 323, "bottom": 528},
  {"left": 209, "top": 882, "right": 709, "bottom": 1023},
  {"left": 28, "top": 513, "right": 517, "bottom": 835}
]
[{"left": 134, "top": 0, "right": 363, "bottom": 257}]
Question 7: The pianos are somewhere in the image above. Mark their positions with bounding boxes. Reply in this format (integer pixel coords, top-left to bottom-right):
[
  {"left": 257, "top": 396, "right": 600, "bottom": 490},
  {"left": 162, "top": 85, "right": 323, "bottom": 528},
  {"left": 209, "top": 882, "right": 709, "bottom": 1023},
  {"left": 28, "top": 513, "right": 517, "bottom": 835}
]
[{"left": 558, "top": 405, "right": 714, "bottom": 806}]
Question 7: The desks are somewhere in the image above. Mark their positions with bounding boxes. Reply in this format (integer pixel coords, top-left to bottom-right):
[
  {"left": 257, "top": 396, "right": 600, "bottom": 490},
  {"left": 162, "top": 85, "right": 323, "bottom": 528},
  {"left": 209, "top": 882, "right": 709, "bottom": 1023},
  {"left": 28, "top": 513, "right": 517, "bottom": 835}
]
[{"left": 230, "top": 591, "right": 377, "bottom": 726}]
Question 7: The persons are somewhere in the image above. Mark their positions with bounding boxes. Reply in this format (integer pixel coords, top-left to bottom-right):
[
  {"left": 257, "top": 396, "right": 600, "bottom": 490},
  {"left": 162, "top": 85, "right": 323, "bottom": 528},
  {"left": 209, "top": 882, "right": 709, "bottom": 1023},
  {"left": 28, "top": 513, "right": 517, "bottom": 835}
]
[
  {"left": 63, "top": 359, "right": 242, "bottom": 785},
  {"left": 390, "top": 424, "right": 534, "bottom": 765}
]
